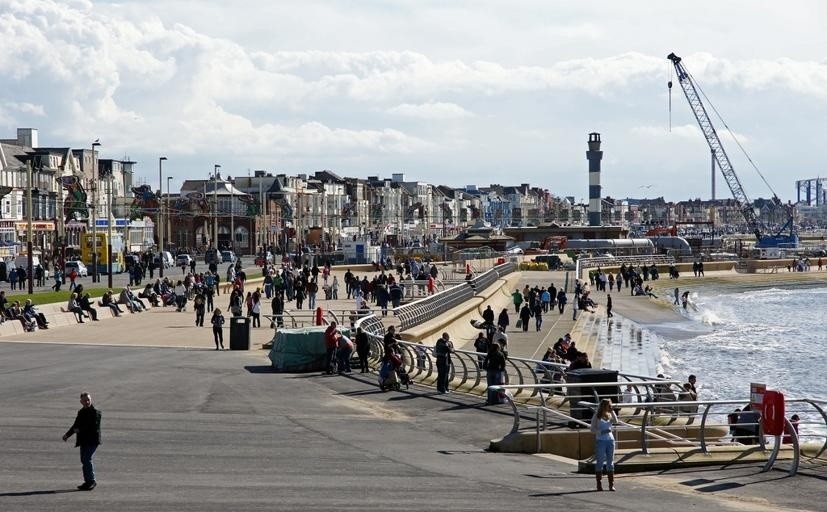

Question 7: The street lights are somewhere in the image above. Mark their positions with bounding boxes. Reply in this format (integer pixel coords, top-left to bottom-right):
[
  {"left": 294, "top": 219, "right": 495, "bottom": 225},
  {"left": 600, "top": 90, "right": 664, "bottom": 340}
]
[
  {"left": 209, "top": 177, "right": 213, "bottom": 240},
  {"left": 166, "top": 176, "right": 173, "bottom": 252},
  {"left": 92, "top": 143, "right": 101, "bottom": 282},
  {"left": 156, "top": 157, "right": 167, "bottom": 251},
  {"left": 56, "top": 164, "right": 65, "bottom": 238},
  {"left": 258, "top": 172, "right": 265, "bottom": 246},
  {"left": 103, "top": 168, "right": 115, "bottom": 288},
  {"left": 230, "top": 181, "right": 235, "bottom": 250},
  {"left": 213, "top": 164, "right": 221, "bottom": 250},
  {"left": 12, "top": 154, "right": 36, "bottom": 295}
]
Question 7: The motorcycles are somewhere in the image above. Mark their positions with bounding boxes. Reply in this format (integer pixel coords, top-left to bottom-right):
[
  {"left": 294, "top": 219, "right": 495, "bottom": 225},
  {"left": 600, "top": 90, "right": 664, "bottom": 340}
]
[{"left": 381, "top": 342, "right": 413, "bottom": 391}]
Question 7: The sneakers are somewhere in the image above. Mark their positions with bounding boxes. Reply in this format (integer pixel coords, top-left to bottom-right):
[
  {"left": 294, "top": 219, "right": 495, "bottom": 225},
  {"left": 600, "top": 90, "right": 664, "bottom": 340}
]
[{"left": 76, "top": 479, "right": 97, "bottom": 490}]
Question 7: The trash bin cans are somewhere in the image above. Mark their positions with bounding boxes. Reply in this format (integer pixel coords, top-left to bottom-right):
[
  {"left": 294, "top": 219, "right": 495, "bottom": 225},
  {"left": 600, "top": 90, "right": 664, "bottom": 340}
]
[
  {"left": 230, "top": 317, "right": 250, "bottom": 349},
  {"left": 568, "top": 369, "right": 619, "bottom": 427}
]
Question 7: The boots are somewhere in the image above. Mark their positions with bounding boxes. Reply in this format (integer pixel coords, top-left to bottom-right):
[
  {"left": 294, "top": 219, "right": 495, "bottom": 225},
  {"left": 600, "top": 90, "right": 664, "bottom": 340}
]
[
  {"left": 596, "top": 471, "right": 603, "bottom": 490},
  {"left": 607, "top": 470, "right": 616, "bottom": 490}
]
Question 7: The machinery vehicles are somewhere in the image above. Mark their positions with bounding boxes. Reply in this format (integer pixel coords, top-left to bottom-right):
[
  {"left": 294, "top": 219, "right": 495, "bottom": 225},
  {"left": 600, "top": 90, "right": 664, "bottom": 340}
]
[{"left": 664, "top": 49, "right": 802, "bottom": 261}]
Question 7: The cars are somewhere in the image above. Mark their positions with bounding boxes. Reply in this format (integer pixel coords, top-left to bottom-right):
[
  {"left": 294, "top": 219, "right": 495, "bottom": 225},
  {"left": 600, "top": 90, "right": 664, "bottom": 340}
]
[
  {"left": 805, "top": 247, "right": 826, "bottom": 258},
  {"left": 445, "top": 235, "right": 617, "bottom": 266},
  {"left": 599, "top": 221, "right": 826, "bottom": 239}
]
